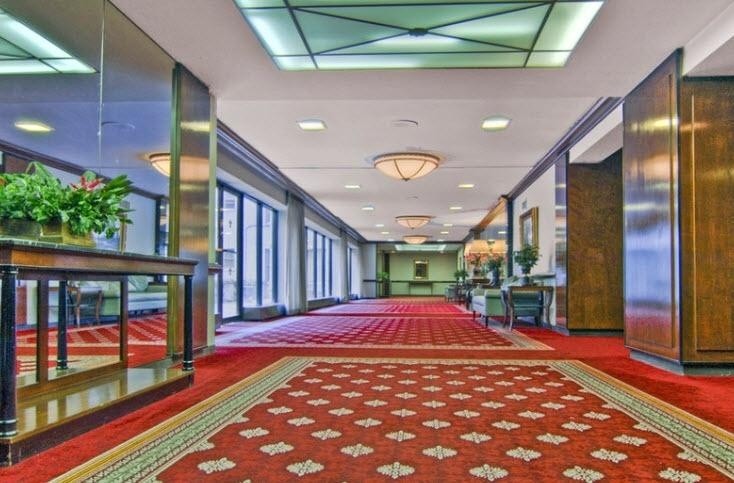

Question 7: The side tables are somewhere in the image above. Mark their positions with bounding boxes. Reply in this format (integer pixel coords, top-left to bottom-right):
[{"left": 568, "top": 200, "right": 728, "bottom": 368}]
[
  {"left": 500, "top": 286, "right": 555, "bottom": 333},
  {"left": 46, "top": 285, "right": 105, "bottom": 328}
]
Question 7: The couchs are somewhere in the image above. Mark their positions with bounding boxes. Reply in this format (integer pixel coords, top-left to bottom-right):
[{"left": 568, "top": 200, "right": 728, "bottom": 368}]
[
  {"left": 470, "top": 275, "right": 544, "bottom": 328},
  {"left": 63, "top": 275, "right": 169, "bottom": 329}
]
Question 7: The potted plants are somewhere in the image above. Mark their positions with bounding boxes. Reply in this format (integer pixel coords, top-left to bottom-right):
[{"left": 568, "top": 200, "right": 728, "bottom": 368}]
[
  {"left": 1, "top": 158, "right": 138, "bottom": 248},
  {"left": 515, "top": 242, "right": 541, "bottom": 285},
  {"left": 487, "top": 256, "right": 503, "bottom": 285}
]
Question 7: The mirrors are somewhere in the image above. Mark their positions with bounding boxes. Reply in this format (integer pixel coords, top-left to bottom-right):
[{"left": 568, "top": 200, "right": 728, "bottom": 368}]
[{"left": 0, "top": 0, "right": 181, "bottom": 410}]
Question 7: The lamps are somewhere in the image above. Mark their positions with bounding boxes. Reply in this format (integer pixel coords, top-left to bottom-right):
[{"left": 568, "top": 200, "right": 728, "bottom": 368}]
[
  {"left": 373, "top": 151, "right": 440, "bottom": 182},
  {"left": 395, "top": 214, "right": 434, "bottom": 246},
  {"left": 465, "top": 237, "right": 491, "bottom": 278},
  {"left": 148, "top": 152, "right": 171, "bottom": 176}
]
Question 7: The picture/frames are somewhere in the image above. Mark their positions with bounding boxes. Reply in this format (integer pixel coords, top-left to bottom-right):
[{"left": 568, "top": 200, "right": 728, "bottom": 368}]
[{"left": 519, "top": 206, "right": 539, "bottom": 248}]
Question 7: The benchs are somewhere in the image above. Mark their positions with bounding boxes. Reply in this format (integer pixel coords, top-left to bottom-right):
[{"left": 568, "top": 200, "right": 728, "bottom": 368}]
[
  {"left": 308, "top": 296, "right": 336, "bottom": 312},
  {"left": 242, "top": 303, "right": 286, "bottom": 321}
]
